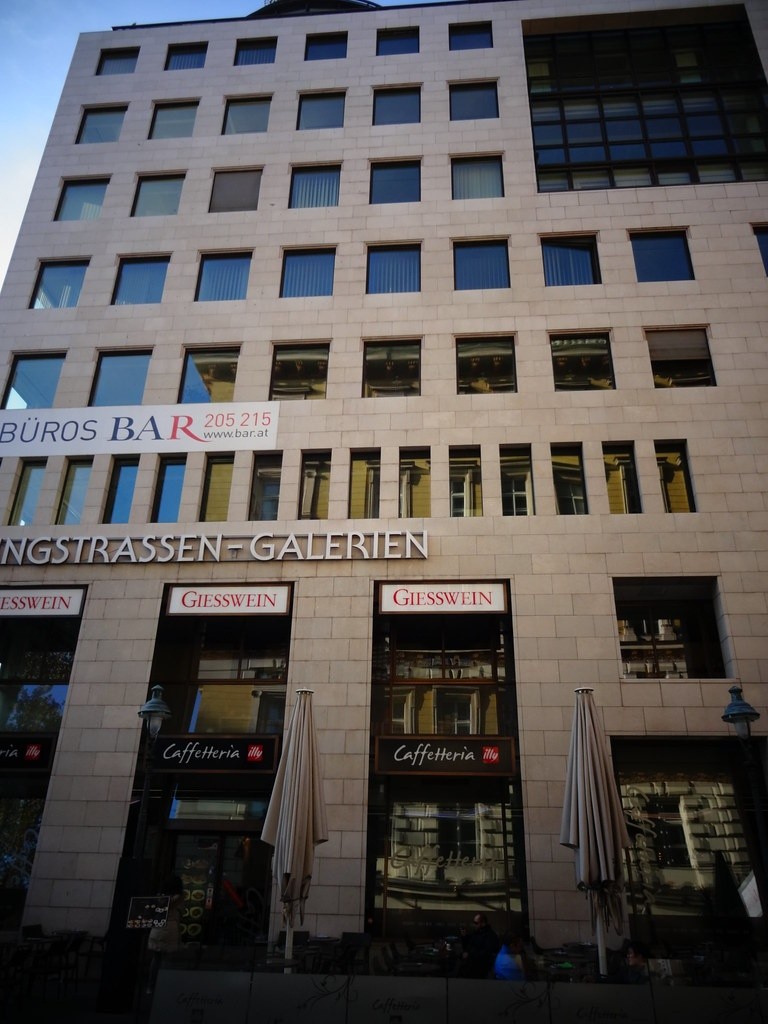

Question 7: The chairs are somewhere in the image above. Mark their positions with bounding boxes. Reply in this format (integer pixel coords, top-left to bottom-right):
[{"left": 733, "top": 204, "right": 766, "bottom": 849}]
[
  {"left": 0, "top": 921, "right": 112, "bottom": 1008},
  {"left": 245, "top": 959, "right": 292, "bottom": 971},
  {"left": 240, "top": 931, "right": 443, "bottom": 979}
]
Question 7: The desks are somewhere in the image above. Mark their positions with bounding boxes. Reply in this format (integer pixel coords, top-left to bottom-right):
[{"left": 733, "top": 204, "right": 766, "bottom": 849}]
[
  {"left": 410, "top": 948, "right": 437, "bottom": 960},
  {"left": 387, "top": 960, "right": 442, "bottom": 979},
  {"left": 288, "top": 946, "right": 320, "bottom": 974},
  {"left": 309, "top": 936, "right": 340, "bottom": 960},
  {"left": 13, "top": 938, "right": 59, "bottom": 991}
]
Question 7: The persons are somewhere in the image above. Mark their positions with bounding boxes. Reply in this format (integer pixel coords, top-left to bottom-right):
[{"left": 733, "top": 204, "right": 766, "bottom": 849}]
[
  {"left": 146, "top": 892, "right": 182, "bottom": 996},
  {"left": 626, "top": 941, "right": 660, "bottom": 984},
  {"left": 464, "top": 912, "right": 498, "bottom": 979},
  {"left": 494, "top": 928, "right": 525, "bottom": 981}
]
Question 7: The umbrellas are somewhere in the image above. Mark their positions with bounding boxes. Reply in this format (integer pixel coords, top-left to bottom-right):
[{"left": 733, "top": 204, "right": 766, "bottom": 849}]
[
  {"left": 558, "top": 687, "right": 633, "bottom": 979},
  {"left": 260, "top": 690, "right": 328, "bottom": 975}
]
